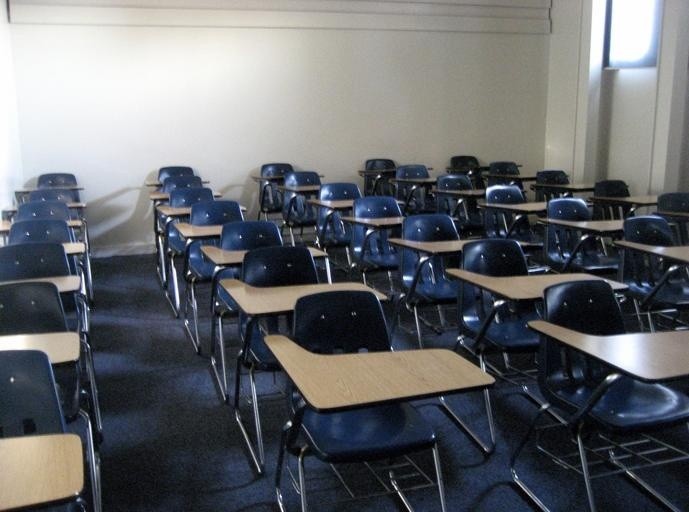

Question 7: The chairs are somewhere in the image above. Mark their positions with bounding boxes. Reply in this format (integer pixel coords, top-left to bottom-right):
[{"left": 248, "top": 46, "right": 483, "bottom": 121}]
[
  {"left": 487, "top": 161, "right": 528, "bottom": 200},
  {"left": 217, "top": 221, "right": 287, "bottom": 348},
  {"left": 29, "top": 187, "right": 75, "bottom": 202},
  {"left": 7, "top": 221, "right": 72, "bottom": 248},
  {"left": 593, "top": 180, "right": 634, "bottom": 219},
  {"left": 158, "top": 167, "right": 192, "bottom": 183},
  {"left": 168, "top": 188, "right": 213, "bottom": 253},
  {"left": 398, "top": 213, "right": 464, "bottom": 331},
  {"left": 2, "top": 349, "right": 102, "bottom": 512},
  {"left": 657, "top": 191, "right": 688, "bottom": 245},
  {"left": 163, "top": 174, "right": 200, "bottom": 192},
  {"left": 283, "top": 171, "right": 322, "bottom": 240},
  {"left": 349, "top": 197, "right": 403, "bottom": 295},
  {"left": 260, "top": 163, "right": 296, "bottom": 223},
  {"left": 620, "top": 215, "right": 689, "bottom": 332},
  {"left": 239, "top": 245, "right": 322, "bottom": 403},
  {"left": 317, "top": 182, "right": 363, "bottom": 262},
  {"left": 533, "top": 280, "right": 687, "bottom": 481},
  {"left": 438, "top": 175, "right": 482, "bottom": 233},
  {"left": 10, "top": 201, "right": 72, "bottom": 224},
  {"left": 37, "top": 174, "right": 77, "bottom": 189},
  {"left": 451, "top": 156, "right": 486, "bottom": 189},
  {"left": 0, "top": 282, "right": 103, "bottom": 443},
  {"left": 543, "top": 199, "right": 619, "bottom": 276},
  {"left": 534, "top": 171, "right": 573, "bottom": 217},
  {"left": 483, "top": 186, "right": 548, "bottom": 269},
  {"left": 365, "top": 158, "right": 396, "bottom": 197},
  {"left": 395, "top": 164, "right": 435, "bottom": 211},
  {"left": 0, "top": 245, "right": 89, "bottom": 334},
  {"left": 293, "top": 291, "right": 435, "bottom": 503},
  {"left": 188, "top": 199, "right": 245, "bottom": 308},
  {"left": 460, "top": 238, "right": 546, "bottom": 377}
]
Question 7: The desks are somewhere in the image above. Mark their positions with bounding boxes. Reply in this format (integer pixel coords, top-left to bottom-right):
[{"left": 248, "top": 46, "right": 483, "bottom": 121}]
[
  {"left": 281, "top": 186, "right": 323, "bottom": 246},
  {"left": 437, "top": 266, "right": 628, "bottom": 456},
  {"left": 588, "top": 194, "right": 658, "bottom": 219},
  {"left": 651, "top": 211, "right": 687, "bottom": 245},
  {"left": 146, "top": 178, "right": 212, "bottom": 185},
  {"left": 538, "top": 216, "right": 675, "bottom": 273},
  {"left": 310, "top": 197, "right": 404, "bottom": 252},
  {"left": 199, "top": 245, "right": 328, "bottom": 405},
  {"left": 360, "top": 167, "right": 434, "bottom": 196},
  {"left": 0, "top": 218, "right": 81, "bottom": 246},
  {"left": 611, "top": 241, "right": 688, "bottom": 332},
  {"left": 214, "top": 279, "right": 388, "bottom": 475},
  {"left": 529, "top": 184, "right": 628, "bottom": 200},
  {"left": 0, "top": 433, "right": 88, "bottom": 512},
  {"left": 508, "top": 319, "right": 689, "bottom": 512},
  {"left": 1, "top": 331, "right": 79, "bottom": 364},
  {"left": 484, "top": 171, "right": 536, "bottom": 185},
  {"left": 341, "top": 215, "right": 460, "bottom": 301},
  {"left": 14, "top": 186, "right": 85, "bottom": 207},
  {"left": 263, "top": 335, "right": 495, "bottom": 512},
  {"left": 253, "top": 175, "right": 323, "bottom": 223},
  {"left": 152, "top": 193, "right": 222, "bottom": 198},
  {"left": 389, "top": 175, "right": 438, "bottom": 214},
  {"left": 1, "top": 201, "right": 87, "bottom": 219},
  {"left": 481, "top": 200, "right": 595, "bottom": 240},
  {"left": 387, "top": 237, "right": 544, "bottom": 334},
  {"left": 1, "top": 275, "right": 83, "bottom": 292},
  {"left": 174, "top": 222, "right": 223, "bottom": 353},
  {"left": 61, "top": 241, "right": 86, "bottom": 253},
  {"left": 446, "top": 164, "right": 522, "bottom": 189},
  {"left": 432, "top": 186, "right": 528, "bottom": 237},
  {"left": 156, "top": 202, "right": 247, "bottom": 286}
]
